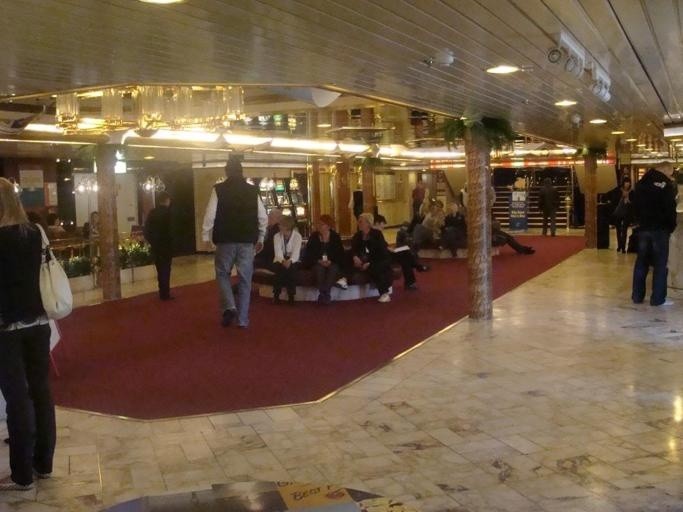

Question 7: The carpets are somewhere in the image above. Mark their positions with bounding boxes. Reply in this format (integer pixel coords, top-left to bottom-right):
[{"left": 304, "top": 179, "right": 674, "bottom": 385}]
[{"left": 47, "top": 235, "right": 587, "bottom": 420}]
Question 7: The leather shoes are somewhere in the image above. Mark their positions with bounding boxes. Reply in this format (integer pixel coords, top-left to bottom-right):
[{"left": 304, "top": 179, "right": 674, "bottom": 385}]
[
  {"left": 522, "top": 247, "right": 536, "bottom": 255},
  {"left": 405, "top": 284, "right": 417, "bottom": 290},
  {"left": 416, "top": 264, "right": 430, "bottom": 272}
]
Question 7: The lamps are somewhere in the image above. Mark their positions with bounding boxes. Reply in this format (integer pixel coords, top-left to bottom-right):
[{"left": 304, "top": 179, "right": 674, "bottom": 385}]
[
  {"left": 137, "top": 175, "right": 166, "bottom": 194},
  {"left": 549, "top": 32, "right": 611, "bottom": 103},
  {"left": 71, "top": 173, "right": 98, "bottom": 199},
  {"left": 57, "top": 85, "right": 245, "bottom": 133}
]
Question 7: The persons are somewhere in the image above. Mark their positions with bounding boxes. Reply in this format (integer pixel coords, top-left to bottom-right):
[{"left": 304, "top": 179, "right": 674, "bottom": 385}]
[
  {"left": 200, "top": 154, "right": 269, "bottom": 328},
  {"left": 47, "top": 211, "right": 65, "bottom": 239},
  {"left": 353, "top": 183, "right": 363, "bottom": 218},
  {"left": 629, "top": 160, "right": 679, "bottom": 306},
  {"left": 142, "top": 191, "right": 178, "bottom": 301},
  {"left": 82, "top": 211, "right": 100, "bottom": 237},
  {"left": 264, "top": 201, "right": 535, "bottom": 306},
  {"left": 411, "top": 183, "right": 424, "bottom": 210},
  {"left": 537, "top": 178, "right": 560, "bottom": 238},
  {"left": 0, "top": 176, "right": 72, "bottom": 491},
  {"left": 601, "top": 176, "right": 632, "bottom": 253}
]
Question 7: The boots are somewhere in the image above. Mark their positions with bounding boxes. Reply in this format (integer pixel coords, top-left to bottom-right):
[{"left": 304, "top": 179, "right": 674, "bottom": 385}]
[
  {"left": 288, "top": 295, "right": 294, "bottom": 305},
  {"left": 272, "top": 293, "right": 281, "bottom": 304}
]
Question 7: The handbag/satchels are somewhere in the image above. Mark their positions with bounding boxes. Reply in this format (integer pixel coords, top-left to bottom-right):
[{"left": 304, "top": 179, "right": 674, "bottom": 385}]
[
  {"left": 627, "top": 227, "right": 639, "bottom": 253},
  {"left": 39, "top": 260, "right": 73, "bottom": 321}
]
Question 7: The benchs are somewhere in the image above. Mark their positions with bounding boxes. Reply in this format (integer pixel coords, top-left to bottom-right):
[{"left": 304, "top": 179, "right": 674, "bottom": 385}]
[
  {"left": 407, "top": 243, "right": 500, "bottom": 258},
  {"left": 253, "top": 240, "right": 393, "bottom": 299}
]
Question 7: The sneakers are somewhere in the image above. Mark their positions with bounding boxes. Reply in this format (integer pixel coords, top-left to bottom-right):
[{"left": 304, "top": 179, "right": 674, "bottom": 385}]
[
  {"left": 663, "top": 296, "right": 674, "bottom": 306},
  {"left": 335, "top": 279, "right": 348, "bottom": 290},
  {"left": 377, "top": 293, "right": 392, "bottom": 303},
  {"left": 222, "top": 309, "right": 237, "bottom": 327},
  {"left": 0, "top": 467, "right": 51, "bottom": 490},
  {"left": 318, "top": 294, "right": 331, "bottom": 305}
]
